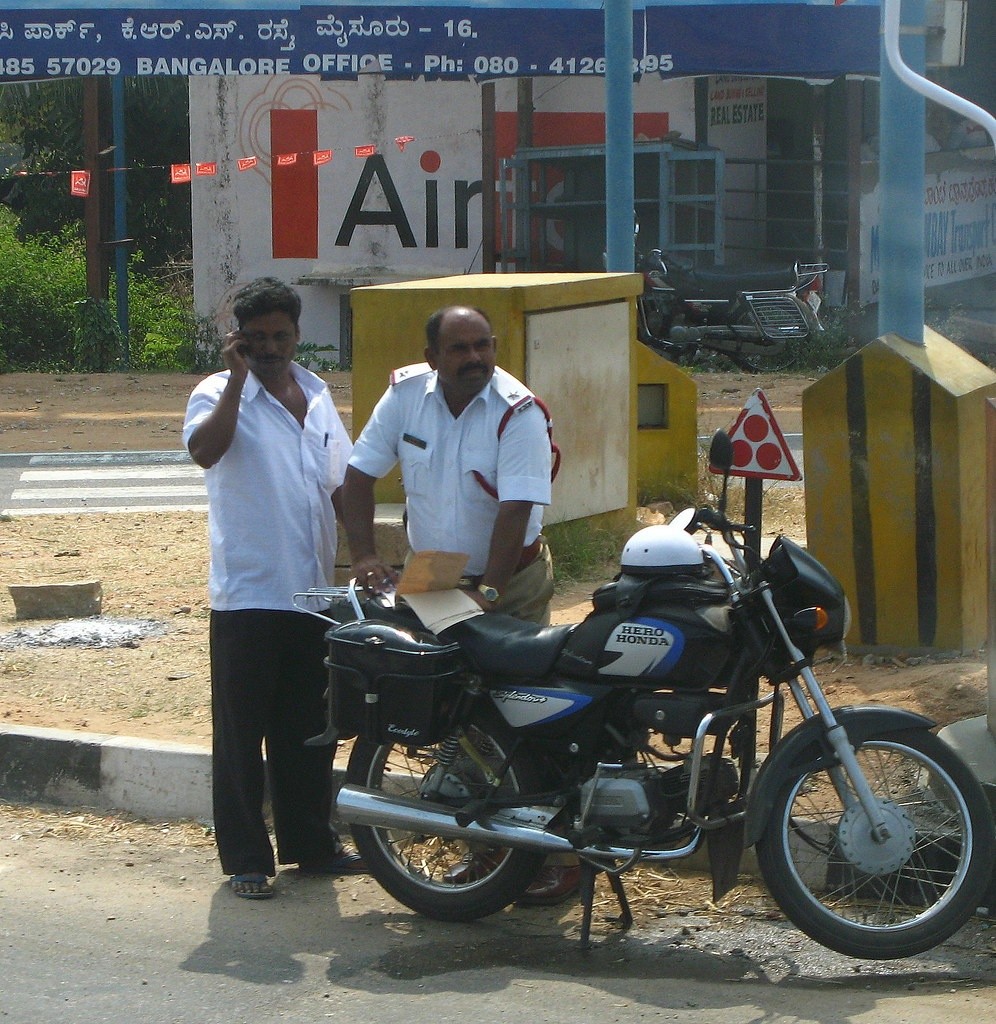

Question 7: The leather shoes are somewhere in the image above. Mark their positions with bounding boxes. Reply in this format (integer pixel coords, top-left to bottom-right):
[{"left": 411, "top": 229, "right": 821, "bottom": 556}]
[
  {"left": 520, "top": 865, "right": 591, "bottom": 904},
  {"left": 442, "top": 847, "right": 513, "bottom": 884}
]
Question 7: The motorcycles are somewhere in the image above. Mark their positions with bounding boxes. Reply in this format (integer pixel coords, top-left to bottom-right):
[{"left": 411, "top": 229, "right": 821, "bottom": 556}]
[
  {"left": 602, "top": 209, "right": 831, "bottom": 375},
  {"left": 291, "top": 423, "right": 995, "bottom": 961}
]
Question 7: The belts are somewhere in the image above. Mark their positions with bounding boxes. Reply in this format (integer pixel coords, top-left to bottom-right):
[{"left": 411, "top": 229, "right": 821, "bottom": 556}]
[{"left": 454, "top": 534, "right": 542, "bottom": 590}]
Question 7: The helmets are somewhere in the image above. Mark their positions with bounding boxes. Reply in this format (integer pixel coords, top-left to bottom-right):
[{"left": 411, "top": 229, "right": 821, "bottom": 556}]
[{"left": 616, "top": 525, "right": 705, "bottom": 577}]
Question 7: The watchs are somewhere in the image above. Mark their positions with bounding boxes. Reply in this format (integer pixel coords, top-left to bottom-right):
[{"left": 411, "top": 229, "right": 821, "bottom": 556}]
[{"left": 477, "top": 584, "right": 502, "bottom": 605}]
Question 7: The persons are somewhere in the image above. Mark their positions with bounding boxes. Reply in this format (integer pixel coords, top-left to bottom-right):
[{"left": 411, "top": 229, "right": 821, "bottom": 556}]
[
  {"left": 182, "top": 278, "right": 398, "bottom": 899},
  {"left": 342, "top": 305, "right": 587, "bottom": 905}
]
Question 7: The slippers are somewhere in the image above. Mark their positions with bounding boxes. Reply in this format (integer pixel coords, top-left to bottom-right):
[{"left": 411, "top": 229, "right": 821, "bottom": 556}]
[
  {"left": 298, "top": 855, "right": 370, "bottom": 875},
  {"left": 230, "top": 874, "right": 273, "bottom": 899}
]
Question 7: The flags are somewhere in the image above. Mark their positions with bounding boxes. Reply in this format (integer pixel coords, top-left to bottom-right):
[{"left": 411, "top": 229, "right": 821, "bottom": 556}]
[
  {"left": 314, "top": 150, "right": 332, "bottom": 166},
  {"left": 71, "top": 171, "right": 90, "bottom": 196},
  {"left": 396, "top": 135, "right": 414, "bottom": 152},
  {"left": 355, "top": 145, "right": 375, "bottom": 156},
  {"left": 237, "top": 157, "right": 256, "bottom": 170},
  {"left": 196, "top": 162, "right": 217, "bottom": 175},
  {"left": 171, "top": 165, "right": 191, "bottom": 183},
  {"left": 278, "top": 153, "right": 296, "bottom": 165}
]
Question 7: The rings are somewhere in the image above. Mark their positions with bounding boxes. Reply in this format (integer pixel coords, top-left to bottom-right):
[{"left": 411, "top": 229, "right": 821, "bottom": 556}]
[
  {"left": 382, "top": 578, "right": 389, "bottom": 584},
  {"left": 367, "top": 572, "right": 374, "bottom": 576}
]
